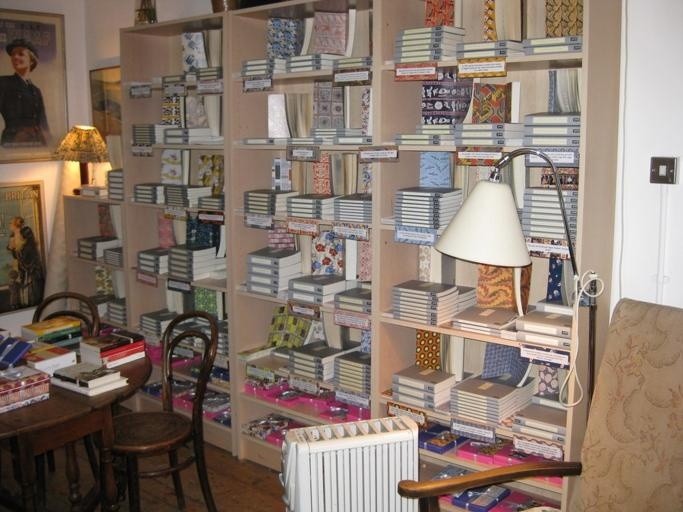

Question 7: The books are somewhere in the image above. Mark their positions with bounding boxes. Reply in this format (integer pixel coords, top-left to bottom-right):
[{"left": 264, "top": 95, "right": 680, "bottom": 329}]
[
  {"left": 387, "top": 0, "right": 582, "bottom": 512},
  {"left": 78, "top": 235, "right": 125, "bottom": 323},
  {"left": 242, "top": 8, "right": 371, "bottom": 396},
  {"left": 81, "top": 169, "right": 124, "bottom": 200},
  {"left": 1, "top": 316, "right": 146, "bottom": 414},
  {"left": 133, "top": 30, "right": 231, "bottom": 428}
]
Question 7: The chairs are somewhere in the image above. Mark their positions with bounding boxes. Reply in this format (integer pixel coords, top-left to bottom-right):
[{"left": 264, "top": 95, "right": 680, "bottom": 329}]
[
  {"left": 109, "top": 310, "right": 221, "bottom": 510},
  {"left": 30, "top": 290, "right": 124, "bottom": 512},
  {"left": 398, "top": 295, "right": 682, "bottom": 512}
]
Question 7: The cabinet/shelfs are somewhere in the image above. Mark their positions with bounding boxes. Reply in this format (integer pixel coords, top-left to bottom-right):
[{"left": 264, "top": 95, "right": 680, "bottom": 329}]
[
  {"left": 63, "top": 192, "right": 125, "bottom": 329},
  {"left": 378, "top": 2, "right": 624, "bottom": 510},
  {"left": 229, "top": 3, "right": 381, "bottom": 477},
  {"left": 120, "top": 12, "right": 227, "bottom": 451}
]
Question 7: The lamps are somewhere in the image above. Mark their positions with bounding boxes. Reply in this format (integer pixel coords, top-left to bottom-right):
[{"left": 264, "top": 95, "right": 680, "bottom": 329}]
[{"left": 48, "top": 124, "right": 112, "bottom": 195}]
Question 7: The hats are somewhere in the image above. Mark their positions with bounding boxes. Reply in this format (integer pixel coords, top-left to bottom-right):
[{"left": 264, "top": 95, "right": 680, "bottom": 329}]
[{"left": 4, "top": 37, "right": 39, "bottom": 58}]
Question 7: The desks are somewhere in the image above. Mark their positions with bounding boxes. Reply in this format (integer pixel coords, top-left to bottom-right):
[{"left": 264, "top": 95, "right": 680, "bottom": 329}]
[{"left": 1, "top": 326, "right": 150, "bottom": 512}]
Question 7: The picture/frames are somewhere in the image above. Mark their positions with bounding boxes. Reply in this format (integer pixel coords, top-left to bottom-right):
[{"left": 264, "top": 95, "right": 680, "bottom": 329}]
[
  {"left": 89, "top": 66, "right": 122, "bottom": 137},
  {"left": 0, "top": 8, "right": 70, "bottom": 163},
  {"left": 0, "top": 177, "right": 47, "bottom": 317}
]
